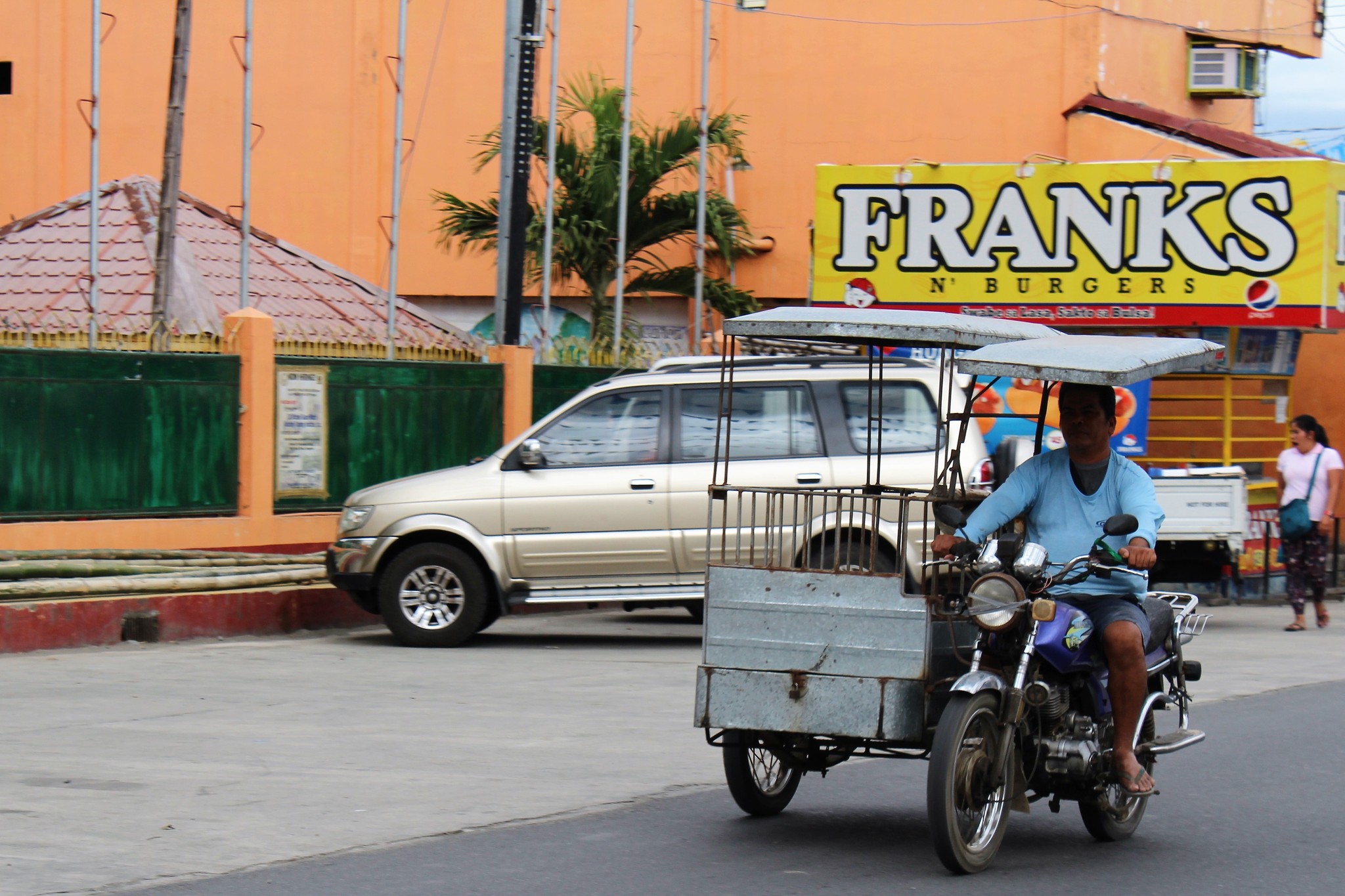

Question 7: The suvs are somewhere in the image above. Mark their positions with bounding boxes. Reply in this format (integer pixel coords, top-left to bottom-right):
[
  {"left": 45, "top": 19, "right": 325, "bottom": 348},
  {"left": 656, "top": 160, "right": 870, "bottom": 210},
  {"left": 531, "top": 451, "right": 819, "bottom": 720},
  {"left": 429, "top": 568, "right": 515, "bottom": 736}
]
[{"left": 324, "top": 356, "right": 999, "bottom": 650}]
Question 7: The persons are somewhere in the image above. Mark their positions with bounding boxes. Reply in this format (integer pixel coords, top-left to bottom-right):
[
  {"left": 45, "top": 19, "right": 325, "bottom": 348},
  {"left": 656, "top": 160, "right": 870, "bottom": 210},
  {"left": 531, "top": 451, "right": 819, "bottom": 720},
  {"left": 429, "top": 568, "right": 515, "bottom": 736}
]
[
  {"left": 1276, "top": 414, "right": 1344, "bottom": 631},
  {"left": 931, "top": 382, "right": 1166, "bottom": 795}
]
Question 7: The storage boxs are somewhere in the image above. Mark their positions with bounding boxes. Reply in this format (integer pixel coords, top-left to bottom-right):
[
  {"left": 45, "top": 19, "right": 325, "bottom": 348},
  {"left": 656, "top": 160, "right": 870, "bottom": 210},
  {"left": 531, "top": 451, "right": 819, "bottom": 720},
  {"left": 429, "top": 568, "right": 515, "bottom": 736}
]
[{"left": 1151, "top": 467, "right": 1250, "bottom": 530}]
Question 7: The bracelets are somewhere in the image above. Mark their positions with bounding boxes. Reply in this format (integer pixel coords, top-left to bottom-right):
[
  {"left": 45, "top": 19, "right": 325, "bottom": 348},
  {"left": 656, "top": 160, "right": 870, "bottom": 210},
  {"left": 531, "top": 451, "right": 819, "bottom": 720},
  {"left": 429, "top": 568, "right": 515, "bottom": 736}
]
[{"left": 1324, "top": 511, "right": 1333, "bottom": 516}]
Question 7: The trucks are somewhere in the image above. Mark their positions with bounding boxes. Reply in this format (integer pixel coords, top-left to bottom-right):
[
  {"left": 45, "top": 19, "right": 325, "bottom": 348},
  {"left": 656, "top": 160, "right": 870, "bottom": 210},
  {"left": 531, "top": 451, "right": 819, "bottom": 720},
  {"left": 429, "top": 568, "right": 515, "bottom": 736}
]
[{"left": 605, "top": 353, "right": 1252, "bottom": 619}]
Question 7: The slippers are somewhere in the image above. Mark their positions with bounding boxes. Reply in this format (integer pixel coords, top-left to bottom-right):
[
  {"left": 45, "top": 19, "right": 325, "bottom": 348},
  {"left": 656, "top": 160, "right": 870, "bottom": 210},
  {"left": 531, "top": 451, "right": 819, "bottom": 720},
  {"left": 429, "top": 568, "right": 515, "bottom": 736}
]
[
  {"left": 1284, "top": 624, "right": 1307, "bottom": 631},
  {"left": 1108, "top": 763, "right": 1156, "bottom": 797},
  {"left": 1317, "top": 609, "right": 1329, "bottom": 628}
]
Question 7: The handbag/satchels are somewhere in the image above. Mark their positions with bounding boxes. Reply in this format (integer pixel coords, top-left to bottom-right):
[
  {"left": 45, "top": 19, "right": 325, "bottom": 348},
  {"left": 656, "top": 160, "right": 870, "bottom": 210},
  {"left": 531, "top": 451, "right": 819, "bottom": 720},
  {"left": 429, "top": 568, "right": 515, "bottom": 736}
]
[{"left": 1278, "top": 499, "right": 1312, "bottom": 540}]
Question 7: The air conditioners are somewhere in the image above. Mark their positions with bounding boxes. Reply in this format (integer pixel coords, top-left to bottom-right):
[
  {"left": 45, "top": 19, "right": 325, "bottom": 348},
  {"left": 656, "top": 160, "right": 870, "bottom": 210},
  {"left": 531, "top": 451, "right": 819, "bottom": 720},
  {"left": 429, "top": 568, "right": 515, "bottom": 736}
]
[{"left": 1189, "top": 45, "right": 1259, "bottom": 94}]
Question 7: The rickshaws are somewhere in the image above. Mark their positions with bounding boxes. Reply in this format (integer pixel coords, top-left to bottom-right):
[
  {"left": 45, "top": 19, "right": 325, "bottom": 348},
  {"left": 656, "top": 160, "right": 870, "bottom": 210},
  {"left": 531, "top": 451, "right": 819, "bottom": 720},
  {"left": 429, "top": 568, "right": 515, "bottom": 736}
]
[{"left": 693, "top": 305, "right": 1226, "bottom": 877}]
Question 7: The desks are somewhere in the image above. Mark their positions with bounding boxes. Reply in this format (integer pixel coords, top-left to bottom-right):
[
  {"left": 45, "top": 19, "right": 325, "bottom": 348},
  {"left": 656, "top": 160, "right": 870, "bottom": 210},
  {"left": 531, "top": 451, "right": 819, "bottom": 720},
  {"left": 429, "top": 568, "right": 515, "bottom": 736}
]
[{"left": 1149, "top": 531, "right": 1244, "bottom": 606}]
